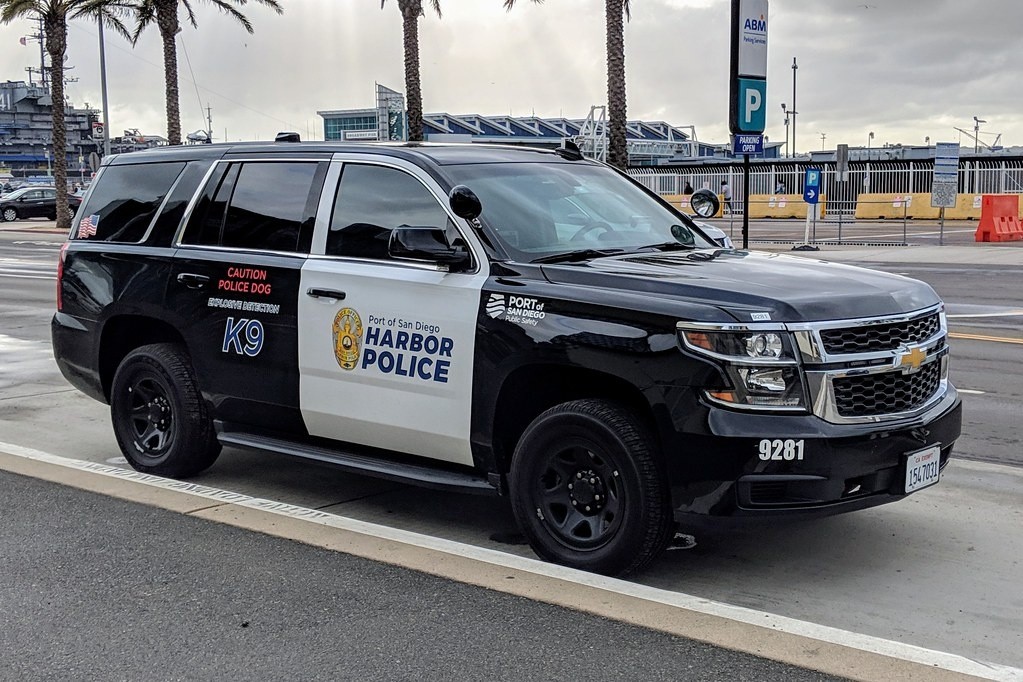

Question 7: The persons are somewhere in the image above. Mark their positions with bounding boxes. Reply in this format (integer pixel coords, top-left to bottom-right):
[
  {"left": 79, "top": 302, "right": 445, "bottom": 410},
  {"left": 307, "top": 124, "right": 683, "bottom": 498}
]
[
  {"left": 684, "top": 181, "right": 694, "bottom": 194},
  {"left": 721, "top": 181, "right": 732, "bottom": 210},
  {"left": 775, "top": 180, "right": 785, "bottom": 194}
]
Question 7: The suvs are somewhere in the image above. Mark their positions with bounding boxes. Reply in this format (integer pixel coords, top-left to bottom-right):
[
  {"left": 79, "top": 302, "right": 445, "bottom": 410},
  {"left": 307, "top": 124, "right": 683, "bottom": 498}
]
[{"left": 49, "top": 132, "right": 968, "bottom": 576}]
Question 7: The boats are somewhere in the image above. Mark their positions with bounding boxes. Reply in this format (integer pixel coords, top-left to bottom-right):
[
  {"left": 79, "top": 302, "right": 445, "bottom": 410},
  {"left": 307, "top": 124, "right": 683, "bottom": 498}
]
[{"left": 0, "top": 40, "right": 171, "bottom": 195}]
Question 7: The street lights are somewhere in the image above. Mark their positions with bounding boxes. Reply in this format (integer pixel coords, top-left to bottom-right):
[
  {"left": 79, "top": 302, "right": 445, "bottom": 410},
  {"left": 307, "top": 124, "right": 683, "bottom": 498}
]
[
  {"left": 792, "top": 55, "right": 799, "bottom": 161},
  {"left": 973, "top": 115, "right": 988, "bottom": 154},
  {"left": 866, "top": 132, "right": 876, "bottom": 147},
  {"left": 779, "top": 103, "right": 800, "bottom": 157}
]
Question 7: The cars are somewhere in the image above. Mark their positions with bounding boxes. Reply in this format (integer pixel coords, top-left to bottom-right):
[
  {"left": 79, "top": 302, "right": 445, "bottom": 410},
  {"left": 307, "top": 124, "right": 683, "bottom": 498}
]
[{"left": 0, "top": 187, "right": 84, "bottom": 223}]
